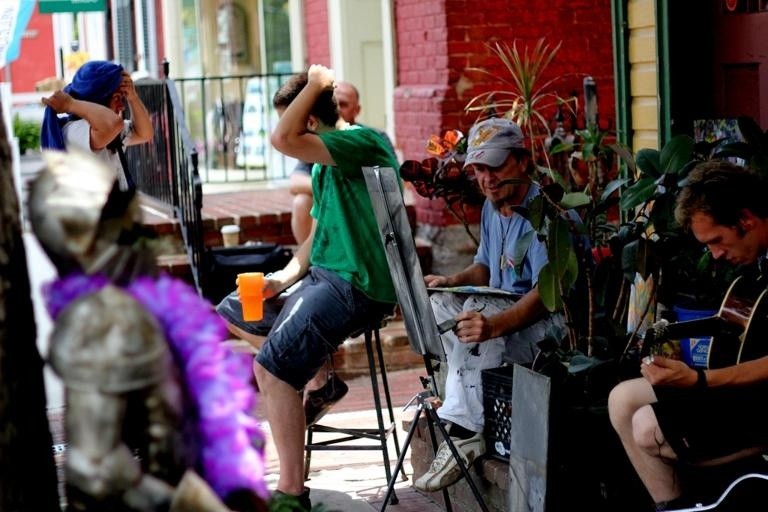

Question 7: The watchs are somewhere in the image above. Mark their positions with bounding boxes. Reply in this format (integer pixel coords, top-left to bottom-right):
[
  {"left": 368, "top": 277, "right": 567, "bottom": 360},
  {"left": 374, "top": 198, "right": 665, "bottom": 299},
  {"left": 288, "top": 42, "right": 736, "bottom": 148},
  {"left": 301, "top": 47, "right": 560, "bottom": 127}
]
[{"left": 694, "top": 369, "right": 707, "bottom": 393}]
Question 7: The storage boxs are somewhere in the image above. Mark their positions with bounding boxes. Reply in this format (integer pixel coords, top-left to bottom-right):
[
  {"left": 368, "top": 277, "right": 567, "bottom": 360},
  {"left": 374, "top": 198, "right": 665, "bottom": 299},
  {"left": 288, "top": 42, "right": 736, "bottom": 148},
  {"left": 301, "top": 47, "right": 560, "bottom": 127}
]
[{"left": 483, "top": 365, "right": 515, "bottom": 466}]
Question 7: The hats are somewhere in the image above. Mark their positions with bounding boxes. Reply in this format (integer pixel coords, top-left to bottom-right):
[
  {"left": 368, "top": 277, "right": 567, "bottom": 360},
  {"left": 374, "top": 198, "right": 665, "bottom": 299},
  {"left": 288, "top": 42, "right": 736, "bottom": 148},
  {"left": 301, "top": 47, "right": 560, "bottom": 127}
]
[{"left": 462, "top": 117, "right": 525, "bottom": 169}]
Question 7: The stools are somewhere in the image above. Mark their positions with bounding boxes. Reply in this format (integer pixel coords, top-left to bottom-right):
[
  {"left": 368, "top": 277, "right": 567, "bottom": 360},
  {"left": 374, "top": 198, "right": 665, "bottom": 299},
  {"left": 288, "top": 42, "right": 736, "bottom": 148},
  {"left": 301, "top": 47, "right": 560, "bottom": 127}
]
[{"left": 303, "top": 319, "right": 408, "bottom": 503}]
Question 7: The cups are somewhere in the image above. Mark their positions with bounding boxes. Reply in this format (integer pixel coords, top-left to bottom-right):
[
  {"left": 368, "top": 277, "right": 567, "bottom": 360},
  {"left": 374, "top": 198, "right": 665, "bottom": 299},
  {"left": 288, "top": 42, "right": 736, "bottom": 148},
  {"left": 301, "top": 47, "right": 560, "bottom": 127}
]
[
  {"left": 221, "top": 225, "right": 240, "bottom": 247},
  {"left": 238, "top": 272, "right": 265, "bottom": 321}
]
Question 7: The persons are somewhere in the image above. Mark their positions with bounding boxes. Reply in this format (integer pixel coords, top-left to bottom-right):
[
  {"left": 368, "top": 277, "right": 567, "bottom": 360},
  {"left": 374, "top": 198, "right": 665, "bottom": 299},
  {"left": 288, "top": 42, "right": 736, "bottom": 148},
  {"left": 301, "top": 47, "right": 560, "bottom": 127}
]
[
  {"left": 42, "top": 61, "right": 152, "bottom": 224},
  {"left": 290, "top": 81, "right": 404, "bottom": 243},
  {"left": 608, "top": 159, "right": 767, "bottom": 509},
  {"left": 415, "top": 117, "right": 591, "bottom": 491},
  {"left": 215, "top": 65, "right": 405, "bottom": 511}
]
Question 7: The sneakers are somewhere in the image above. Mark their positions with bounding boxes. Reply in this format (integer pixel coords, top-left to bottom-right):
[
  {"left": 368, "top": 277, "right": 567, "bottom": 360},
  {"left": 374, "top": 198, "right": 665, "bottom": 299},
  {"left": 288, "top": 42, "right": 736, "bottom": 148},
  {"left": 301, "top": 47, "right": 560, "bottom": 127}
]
[
  {"left": 413, "top": 429, "right": 486, "bottom": 493},
  {"left": 304, "top": 372, "right": 348, "bottom": 425},
  {"left": 273, "top": 487, "right": 312, "bottom": 511}
]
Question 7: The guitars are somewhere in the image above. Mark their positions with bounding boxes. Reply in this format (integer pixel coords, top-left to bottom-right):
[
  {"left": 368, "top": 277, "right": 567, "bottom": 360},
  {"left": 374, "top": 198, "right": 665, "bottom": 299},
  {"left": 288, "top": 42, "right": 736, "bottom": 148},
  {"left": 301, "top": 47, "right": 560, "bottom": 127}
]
[{"left": 641, "top": 275, "right": 768, "bottom": 371}]
